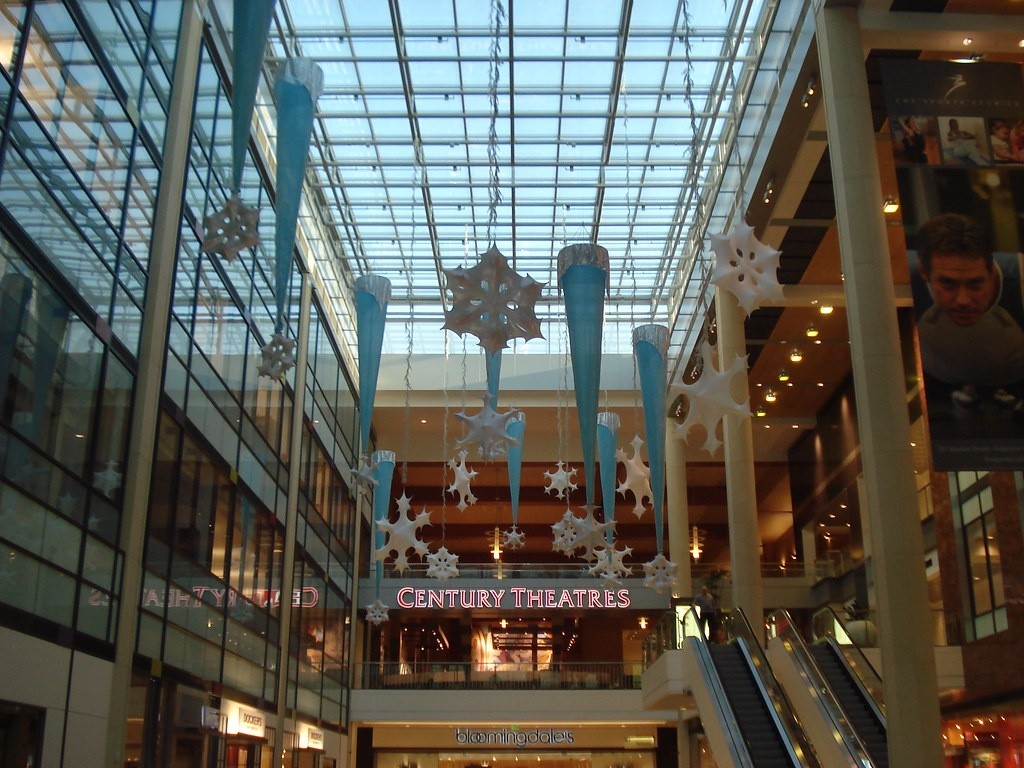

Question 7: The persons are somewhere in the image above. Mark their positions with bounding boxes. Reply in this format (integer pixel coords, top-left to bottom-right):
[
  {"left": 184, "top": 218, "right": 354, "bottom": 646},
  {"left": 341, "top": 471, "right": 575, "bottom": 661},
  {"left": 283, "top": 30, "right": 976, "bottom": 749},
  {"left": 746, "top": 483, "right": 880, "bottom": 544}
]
[
  {"left": 912, "top": 212, "right": 1023, "bottom": 417},
  {"left": 890, "top": 111, "right": 1023, "bottom": 170}
]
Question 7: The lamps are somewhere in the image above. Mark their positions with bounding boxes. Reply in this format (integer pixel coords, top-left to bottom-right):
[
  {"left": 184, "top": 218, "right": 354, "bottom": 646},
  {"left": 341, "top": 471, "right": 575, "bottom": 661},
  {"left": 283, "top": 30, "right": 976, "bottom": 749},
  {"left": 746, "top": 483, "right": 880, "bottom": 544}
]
[
  {"left": 764, "top": 192, "right": 771, "bottom": 203},
  {"left": 778, "top": 369, "right": 790, "bottom": 381},
  {"left": 766, "top": 179, "right": 776, "bottom": 194},
  {"left": 691, "top": 372, "right": 696, "bottom": 379},
  {"left": 807, "top": 323, "right": 818, "bottom": 336},
  {"left": 710, "top": 327, "right": 715, "bottom": 333},
  {"left": 485, "top": 465, "right": 506, "bottom": 559},
  {"left": 757, "top": 406, "right": 766, "bottom": 416},
  {"left": 500, "top": 619, "right": 509, "bottom": 629},
  {"left": 711, "top": 318, "right": 716, "bottom": 326},
  {"left": 675, "top": 410, "right": 681, "bottom": 416},
  {"left": 807, "top": 76, "right": 816, "bottom": 95},
  {"left": 883, "top": 196, "right": 899, "bottom": 212},
  {"left": 766, "top": 389, "right": 776, "bottom": 402},
  {"left": 791, "top": 349, "right": 802, "bottom": 361},
  {"left": 802, "top": 94, "right": 809, "bottom": 108},
  {"left": 690, "top": 501, "right": 706, "bottom": 558},
  {"left": 693, "top": 364, "right": 699, "bottom": 375},
  {"left": 639, "top": 616, "right": 649, "bottom": 629},
  {"left": 493, "top": 559, "right": 507, "bottom": 581},
  {"left": 678, "top": 403, "right": 682, "bottom": 410}
]
